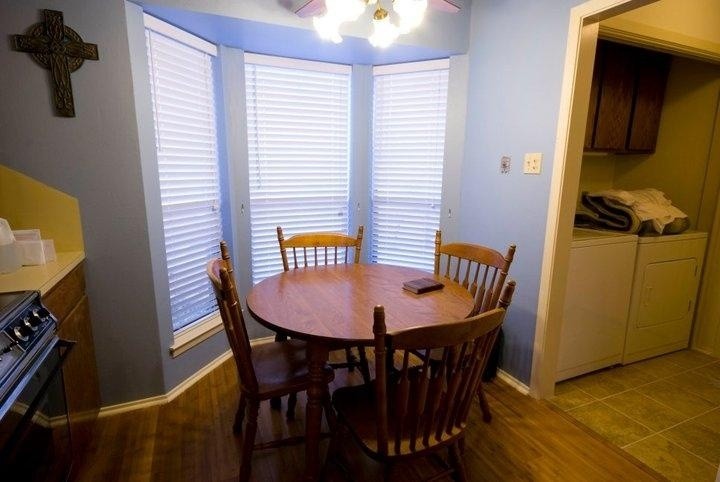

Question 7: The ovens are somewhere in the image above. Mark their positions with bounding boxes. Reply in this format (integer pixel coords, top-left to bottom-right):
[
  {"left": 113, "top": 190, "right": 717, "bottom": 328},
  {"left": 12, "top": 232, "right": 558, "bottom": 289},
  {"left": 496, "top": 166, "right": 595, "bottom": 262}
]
[{"left": 1, "top": 333, "right": 78, "bottom": 480}]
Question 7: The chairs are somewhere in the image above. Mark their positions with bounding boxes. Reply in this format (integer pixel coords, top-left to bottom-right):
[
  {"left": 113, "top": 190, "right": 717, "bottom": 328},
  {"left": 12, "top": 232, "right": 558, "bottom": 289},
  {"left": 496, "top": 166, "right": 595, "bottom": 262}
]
[
  {"left": 275, "top": 225, "right": 378, "bottom": 421},
  {"left": 332, "top": 279, "right": 515, "bottom": 477},
  {"left": 207, "top": 241, "right": 336, "bottom": 479},
  {"left": 403, "top": 227, "right": 516, "bottom": 425}
]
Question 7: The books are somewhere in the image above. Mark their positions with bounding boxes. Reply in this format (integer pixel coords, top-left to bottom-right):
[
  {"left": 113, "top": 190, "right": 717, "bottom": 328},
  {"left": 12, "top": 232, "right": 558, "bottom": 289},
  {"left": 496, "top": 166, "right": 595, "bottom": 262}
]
[{"left": 403, "top": 277, "right": 444, "bottom": 294}]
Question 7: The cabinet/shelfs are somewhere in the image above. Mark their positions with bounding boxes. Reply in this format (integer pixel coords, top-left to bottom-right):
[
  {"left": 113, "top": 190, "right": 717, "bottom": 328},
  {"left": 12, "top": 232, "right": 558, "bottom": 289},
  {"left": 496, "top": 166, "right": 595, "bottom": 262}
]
[{"left": 35, "top": 258, "right": 102, "bottom": 463}]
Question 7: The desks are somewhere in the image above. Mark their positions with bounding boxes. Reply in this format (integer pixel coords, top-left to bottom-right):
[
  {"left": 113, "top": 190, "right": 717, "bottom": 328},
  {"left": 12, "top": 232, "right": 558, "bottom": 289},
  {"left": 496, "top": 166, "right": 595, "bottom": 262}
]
[{"left": 245, "top": 263, "right": 476, "bottom": 482}]
